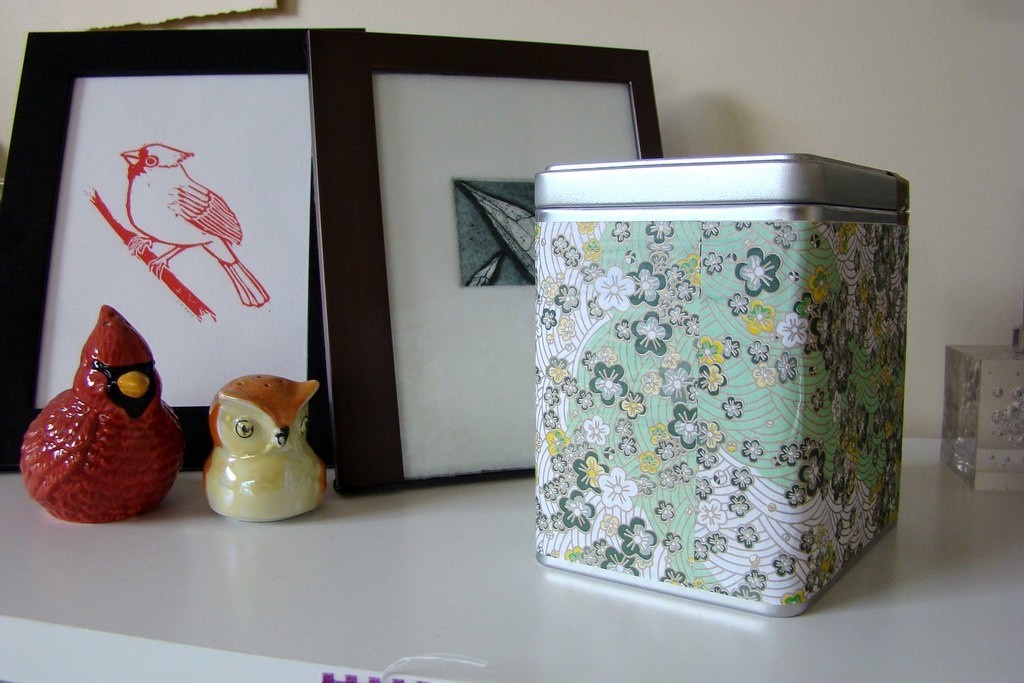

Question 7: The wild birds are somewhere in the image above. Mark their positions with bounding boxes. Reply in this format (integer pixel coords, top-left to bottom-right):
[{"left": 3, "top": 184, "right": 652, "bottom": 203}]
[{"left": 21, "top": 303, "right": 180, "bottom": 523}]
